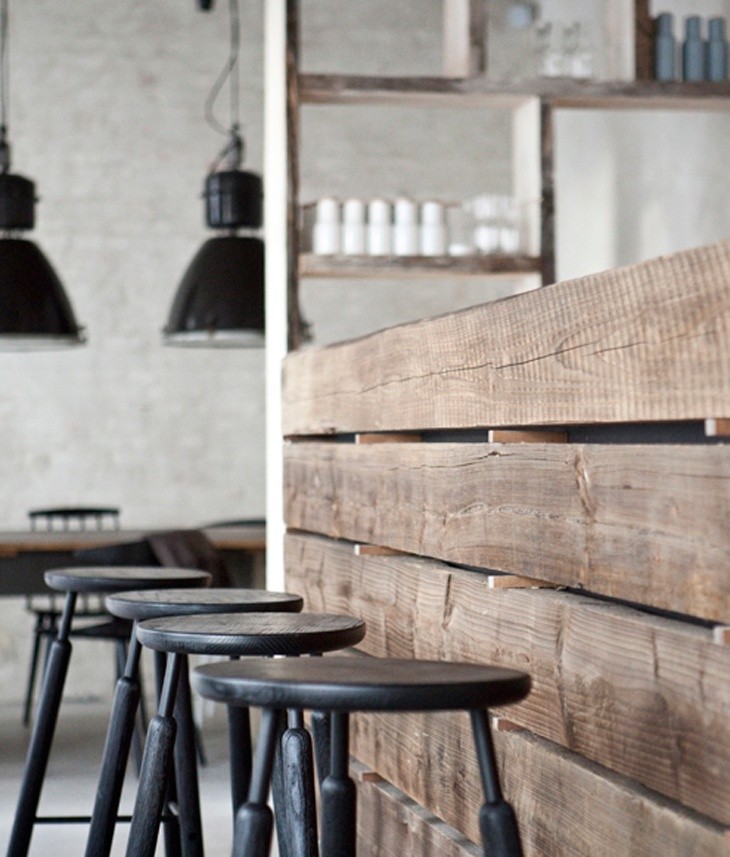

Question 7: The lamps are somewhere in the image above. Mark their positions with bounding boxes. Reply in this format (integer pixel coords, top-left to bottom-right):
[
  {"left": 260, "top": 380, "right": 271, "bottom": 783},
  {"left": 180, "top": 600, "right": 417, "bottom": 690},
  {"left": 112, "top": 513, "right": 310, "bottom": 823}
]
[
  {"left": 0, "top": 0, "right": 97, "bottom": 347},
  {"left": 160, "top": 0, "right": 322, "bottom": 349}
]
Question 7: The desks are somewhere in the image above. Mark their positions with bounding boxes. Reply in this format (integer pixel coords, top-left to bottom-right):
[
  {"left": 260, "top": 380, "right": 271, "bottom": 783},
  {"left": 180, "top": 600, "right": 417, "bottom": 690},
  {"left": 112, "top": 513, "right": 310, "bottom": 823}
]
[{"left": 0, "top": 528, "right": 266, "bottom": 596}]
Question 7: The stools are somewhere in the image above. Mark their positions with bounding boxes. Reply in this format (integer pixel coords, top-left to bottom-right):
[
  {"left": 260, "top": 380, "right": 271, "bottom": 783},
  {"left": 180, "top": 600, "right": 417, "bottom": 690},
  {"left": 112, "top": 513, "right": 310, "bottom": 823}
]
[
  {"left": 5, "top": 567, "right": 215, "bottom": 857},
  {"left": 124, "top": 611, "right": 367, "bottom": 857},
  {"left": 84, "top": 588, "right": 305, "bottom": 857},
  {"left": 191, "top": 657, "right": 536, "bottom": 857}
]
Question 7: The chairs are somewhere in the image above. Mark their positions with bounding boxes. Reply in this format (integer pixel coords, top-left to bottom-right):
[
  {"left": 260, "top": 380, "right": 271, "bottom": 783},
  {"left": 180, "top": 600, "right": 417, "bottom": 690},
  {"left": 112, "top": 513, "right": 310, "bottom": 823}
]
[{"left": 22, "top": 507, "right": 123, "bottom": 725}]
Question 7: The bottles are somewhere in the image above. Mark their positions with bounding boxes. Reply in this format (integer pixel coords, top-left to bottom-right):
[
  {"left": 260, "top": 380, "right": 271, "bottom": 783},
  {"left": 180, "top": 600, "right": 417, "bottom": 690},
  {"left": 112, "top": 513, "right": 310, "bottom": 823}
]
[
  {"left": 653, "top": 13, "right": 730, "bottom": 84},
  {"left": 340, "top": 199, "right": 368, "bottom": 257},
  {"left": 368, "top": 200, "right": 393, "bottom": 258},
  {"left": 419, "top": 202, "right": 449, "bottom": 257},
  {"left": 311, "top": 198, "right": 341, "bottom": 257},
  {"left": 393, "top": 200, "right": 417, "bottom": 258}
]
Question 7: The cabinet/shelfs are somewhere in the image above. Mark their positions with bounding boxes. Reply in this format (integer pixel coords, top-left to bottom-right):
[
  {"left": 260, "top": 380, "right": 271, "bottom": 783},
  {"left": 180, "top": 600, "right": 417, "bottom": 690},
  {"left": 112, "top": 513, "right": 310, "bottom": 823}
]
[{"left": 265, "top": 1, "right": 730, "bottom": 857}]
